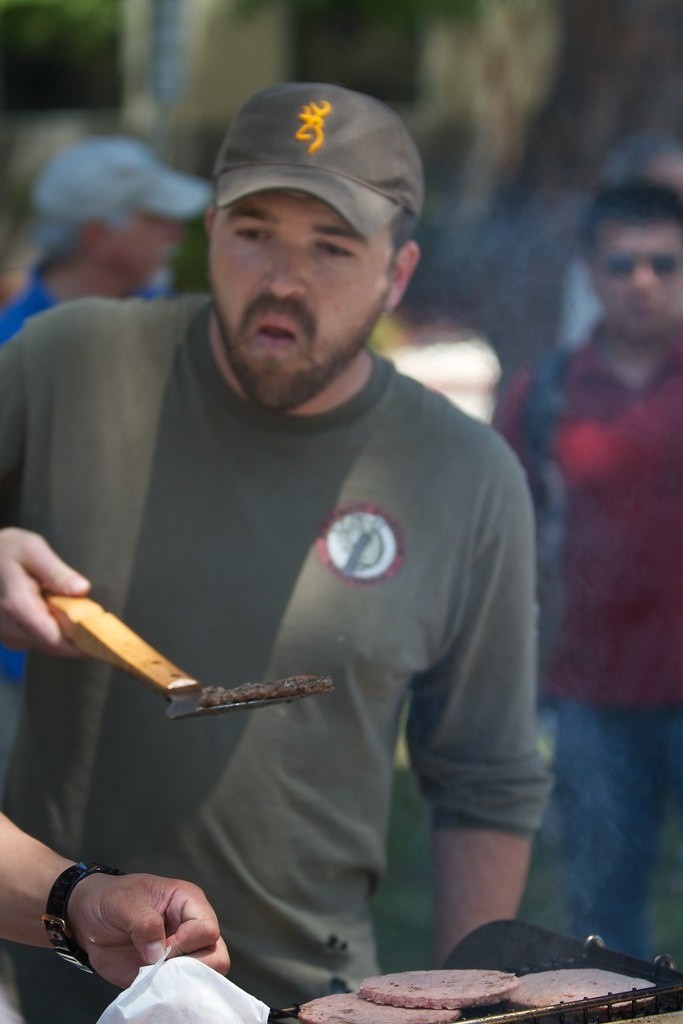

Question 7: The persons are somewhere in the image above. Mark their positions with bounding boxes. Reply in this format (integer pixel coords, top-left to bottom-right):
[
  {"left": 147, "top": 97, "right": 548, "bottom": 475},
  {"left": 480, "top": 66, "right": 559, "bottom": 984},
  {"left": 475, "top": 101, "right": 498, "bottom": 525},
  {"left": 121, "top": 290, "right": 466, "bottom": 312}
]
[
  {"left": 0, "top": 812, "right": 231, "bottom": 992},
  {"left": 0, "top": 138, "right": 683, "bottom": 957},
  {"left": 1, "top": 81, "right": 557, "bottom": 1024}
]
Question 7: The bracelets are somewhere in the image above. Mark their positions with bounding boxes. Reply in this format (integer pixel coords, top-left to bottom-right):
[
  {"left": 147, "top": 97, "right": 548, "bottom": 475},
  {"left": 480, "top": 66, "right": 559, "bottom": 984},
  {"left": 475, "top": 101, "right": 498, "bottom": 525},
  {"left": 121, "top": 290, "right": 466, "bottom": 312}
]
[{"left": 40, "top": 859, "right": 127, "bottom": 974}]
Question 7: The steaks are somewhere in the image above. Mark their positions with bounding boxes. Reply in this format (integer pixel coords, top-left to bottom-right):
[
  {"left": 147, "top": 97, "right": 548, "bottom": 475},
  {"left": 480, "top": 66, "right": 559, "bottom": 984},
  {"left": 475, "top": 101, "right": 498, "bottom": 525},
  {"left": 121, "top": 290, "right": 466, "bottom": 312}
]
[{"left": 198, "top": 674, "right": 334, "bottom": 706}]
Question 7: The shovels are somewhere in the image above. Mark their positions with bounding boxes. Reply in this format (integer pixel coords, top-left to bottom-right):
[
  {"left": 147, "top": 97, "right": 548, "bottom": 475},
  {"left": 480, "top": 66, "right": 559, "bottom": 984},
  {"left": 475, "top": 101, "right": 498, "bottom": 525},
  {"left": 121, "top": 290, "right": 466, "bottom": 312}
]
[{"left": 47, "top": 597, "right": 295, "bottom": 716}]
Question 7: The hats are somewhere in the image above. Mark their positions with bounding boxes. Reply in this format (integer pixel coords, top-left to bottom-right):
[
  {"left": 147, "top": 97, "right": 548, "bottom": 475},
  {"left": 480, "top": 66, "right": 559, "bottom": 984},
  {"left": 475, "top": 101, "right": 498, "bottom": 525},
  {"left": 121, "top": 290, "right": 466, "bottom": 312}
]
[
  {"left": 36, "top": 136, "right": 215, "bottom": 227},
  {"left": 213, "top": 82, "right": 424, "bottom": 238}
]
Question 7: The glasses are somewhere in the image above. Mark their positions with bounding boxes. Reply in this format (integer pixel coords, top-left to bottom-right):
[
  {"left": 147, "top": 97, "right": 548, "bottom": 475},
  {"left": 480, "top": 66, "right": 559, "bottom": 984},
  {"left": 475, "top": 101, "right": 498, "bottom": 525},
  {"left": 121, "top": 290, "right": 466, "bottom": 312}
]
[{"left": 602, "top": 252, "right": 676, "bottom": 276}]
